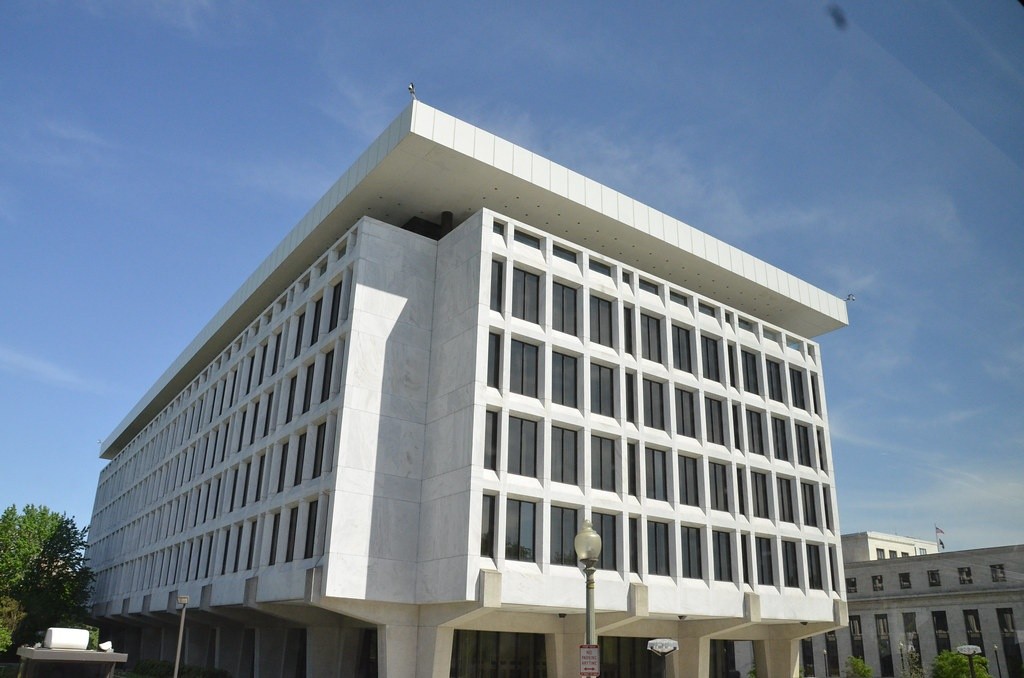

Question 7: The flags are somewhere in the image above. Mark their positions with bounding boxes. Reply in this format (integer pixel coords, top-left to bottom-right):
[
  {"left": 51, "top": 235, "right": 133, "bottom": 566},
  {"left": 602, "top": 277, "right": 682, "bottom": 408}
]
[
  {"left": 938, "top": 538, "right": 945, "bottom": 549},
  {"left": 936, "top": 526, "right": 945, "bottom": 534}
]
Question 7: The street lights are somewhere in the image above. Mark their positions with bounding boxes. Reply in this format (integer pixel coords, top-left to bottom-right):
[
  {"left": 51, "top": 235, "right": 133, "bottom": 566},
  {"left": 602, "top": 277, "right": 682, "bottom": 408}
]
[
  {"left": 994, "top": 644, "right": 1003, "bottom": 678},
  {"left": 823, "top": 648, "right": 828, "bottom": 678},
  {"left": 898, "top": 641, "right": 906, "bottom": 678},
  {"left": 173, "top": 596, "right": 189, "bottom": 678},
  {"left": 574, "top": 520, "right": 602, "bottom": 645}
]
